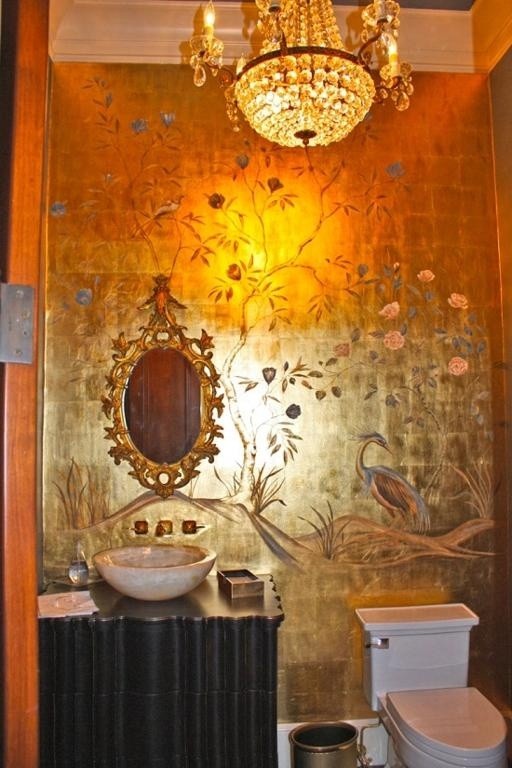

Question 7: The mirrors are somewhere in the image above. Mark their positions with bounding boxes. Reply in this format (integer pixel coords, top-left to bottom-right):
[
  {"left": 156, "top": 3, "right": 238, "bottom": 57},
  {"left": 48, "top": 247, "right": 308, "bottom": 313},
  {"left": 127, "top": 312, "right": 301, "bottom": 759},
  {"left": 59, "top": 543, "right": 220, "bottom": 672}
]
[{"left": 99, "top": 273, "right": 226, "bottom": 499}]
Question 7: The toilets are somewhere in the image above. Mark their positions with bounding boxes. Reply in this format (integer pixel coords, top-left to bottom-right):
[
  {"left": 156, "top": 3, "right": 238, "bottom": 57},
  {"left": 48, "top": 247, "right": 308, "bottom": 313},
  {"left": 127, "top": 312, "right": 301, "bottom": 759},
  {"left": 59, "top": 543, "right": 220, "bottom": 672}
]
[{"left": 357, "top": 605, "right": 503, "bottom": 763}]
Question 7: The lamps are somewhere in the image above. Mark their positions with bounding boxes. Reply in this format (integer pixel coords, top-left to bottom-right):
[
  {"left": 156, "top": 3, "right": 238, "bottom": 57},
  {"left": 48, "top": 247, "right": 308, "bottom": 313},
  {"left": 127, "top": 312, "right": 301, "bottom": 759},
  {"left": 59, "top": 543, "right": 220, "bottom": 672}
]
[{"left": 179, "top": 0, "right": 416, "bottom": 147}]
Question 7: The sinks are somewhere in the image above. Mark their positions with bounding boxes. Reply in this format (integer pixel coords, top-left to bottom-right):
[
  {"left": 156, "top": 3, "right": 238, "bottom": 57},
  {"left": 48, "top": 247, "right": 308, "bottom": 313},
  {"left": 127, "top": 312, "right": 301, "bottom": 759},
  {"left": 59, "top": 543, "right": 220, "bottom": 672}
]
[{"left": 95, "top": 545, "right": 214, "bottom": 600}]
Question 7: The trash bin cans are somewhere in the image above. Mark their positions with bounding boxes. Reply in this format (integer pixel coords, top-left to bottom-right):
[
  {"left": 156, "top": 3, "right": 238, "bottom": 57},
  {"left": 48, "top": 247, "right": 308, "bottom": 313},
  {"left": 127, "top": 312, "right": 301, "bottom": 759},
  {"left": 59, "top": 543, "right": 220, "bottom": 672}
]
[{"left": 288, "top": 722, "right": 359, "bottom": 768}]
